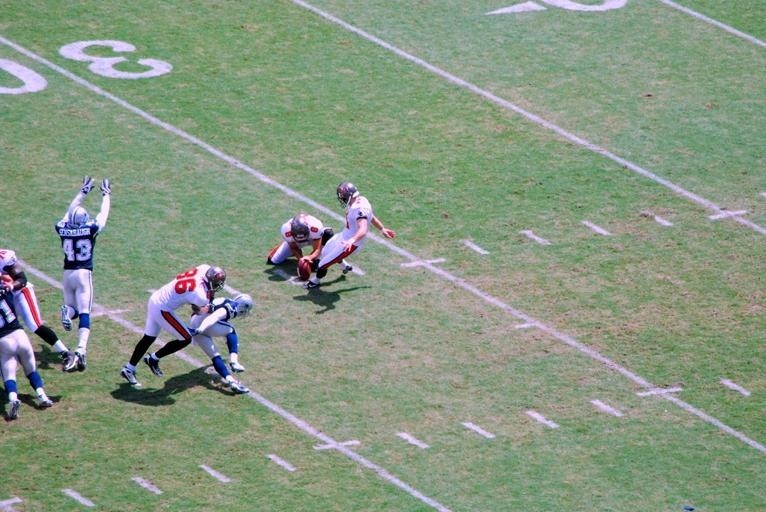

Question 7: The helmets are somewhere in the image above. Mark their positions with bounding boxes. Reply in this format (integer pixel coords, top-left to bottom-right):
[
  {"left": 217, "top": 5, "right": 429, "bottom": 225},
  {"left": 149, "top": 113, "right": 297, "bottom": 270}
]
[
  {"left": 337, "top": 182, "right": 360, "bottom": 209},
  {"left": 233, "top": 293, "right": 254, "bottom": 318},
  {"left": 290, "top": 213, "right": 311, "bottom": 243},
  {"left": 203, "top": 266, "right": 226, "bottom": 292},
  {"left": 67, "top": 205, "right": 89, "bottom": 229}
]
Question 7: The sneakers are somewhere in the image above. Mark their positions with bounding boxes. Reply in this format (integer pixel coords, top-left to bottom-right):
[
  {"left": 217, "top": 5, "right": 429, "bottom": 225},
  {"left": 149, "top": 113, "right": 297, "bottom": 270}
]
[
  {"left": 8, "top": 399, "right": 21, "bottom": 420},
  {"left": 342, "top": 264, "right": 353, "bottom": 275},
  {"left": 143, "top": 352, "right": 164, "bottom": 377},
  {"left": 228, "top": 361, "right": 246, "bottom": 373},
  {"left": 74, "top": 346, "right": 87, "bottom": 372},
  {"left": 39, "top": 395, "right": 54, "bottom": 408},
  {"left": 228, "top": 380, "right": 250, "bottom": 394},
  {"left": 119, "top": 365, "right": 142, "bottom": 387},
  {"left": 60, "top": 304, "right": 74, "bottom": 332},
  {"left": 302, "top": 279, "right": 321, "bottom": 289},
  {"left": 59, "top": 348, "right": 80, "bottom": 371}
]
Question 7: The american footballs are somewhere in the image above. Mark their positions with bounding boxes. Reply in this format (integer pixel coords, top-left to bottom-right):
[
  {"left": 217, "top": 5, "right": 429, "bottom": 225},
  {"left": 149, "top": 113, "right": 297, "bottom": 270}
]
[{"left": 297, "top": 259, "right": 311, "bottom": 280}]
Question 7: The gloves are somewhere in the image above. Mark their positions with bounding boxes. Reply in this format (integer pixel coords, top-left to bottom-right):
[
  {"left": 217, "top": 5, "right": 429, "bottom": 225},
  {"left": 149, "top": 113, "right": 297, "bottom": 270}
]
[
  {"left": 340, "top": 238, "right": 355, "bottom": 253},
  {"left": 187, "top": 327, "right": 202, "bottom": 338},
  {"left": 0, "top": 281, "right": 14, "bottom": 299},
  {"left": 81, "top": 176, "right": 96, "bottom": 195},
  {"left": 99, "top": 178, "right": 112, "bottom": 195},
  {"left": 300, "top": 255, "right": 314, "bottom": 264},
  {"left": 379, "top": 228, "right": 396, "bottom": 239}
]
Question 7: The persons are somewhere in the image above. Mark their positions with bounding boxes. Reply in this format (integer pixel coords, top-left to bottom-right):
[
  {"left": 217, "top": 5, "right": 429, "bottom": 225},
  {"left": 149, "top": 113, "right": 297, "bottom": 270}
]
[
  {"left": 119, "top": 263, "right": 225, "bottom": 386},
  {"left": 1, "top": 274, "right": 54, "bottom": 420},
  {"left": 0, "top": 246, "right": 79, "bottom": 372},
  {"left": 189, "top": 293, "right": 255, "bottom": 395},
  {"left": 52, "top": 174, "right": 113, "bottom": 372},
  {"left": 267, "top": 212, "right": 335, "bottom": 271},
  {"left": 301, "top": 178, "right": 394, "bottom": 290}
]
